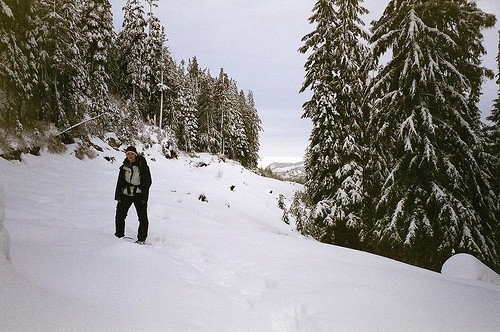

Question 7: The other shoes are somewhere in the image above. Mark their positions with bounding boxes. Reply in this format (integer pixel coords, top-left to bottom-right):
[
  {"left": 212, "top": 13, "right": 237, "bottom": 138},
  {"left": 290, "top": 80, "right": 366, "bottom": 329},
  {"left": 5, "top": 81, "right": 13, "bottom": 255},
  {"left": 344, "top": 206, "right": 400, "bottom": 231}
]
[{"left": 135, "top": 239, "right": 145, "bottom": 244}]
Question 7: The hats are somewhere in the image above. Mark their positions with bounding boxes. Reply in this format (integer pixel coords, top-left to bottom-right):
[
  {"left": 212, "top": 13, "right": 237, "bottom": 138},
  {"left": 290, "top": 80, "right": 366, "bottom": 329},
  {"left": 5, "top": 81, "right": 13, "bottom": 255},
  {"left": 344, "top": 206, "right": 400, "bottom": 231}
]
[{"left": 126, "top": 146, "right": 138, "bottom": 157}]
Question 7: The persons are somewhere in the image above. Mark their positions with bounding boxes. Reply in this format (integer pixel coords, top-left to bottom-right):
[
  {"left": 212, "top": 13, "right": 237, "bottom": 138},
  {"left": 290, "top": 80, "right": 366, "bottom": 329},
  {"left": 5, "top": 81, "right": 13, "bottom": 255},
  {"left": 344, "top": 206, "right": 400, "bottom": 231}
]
[{"left": 114, "top": 146, "right": 152, "bottom": 245}]
